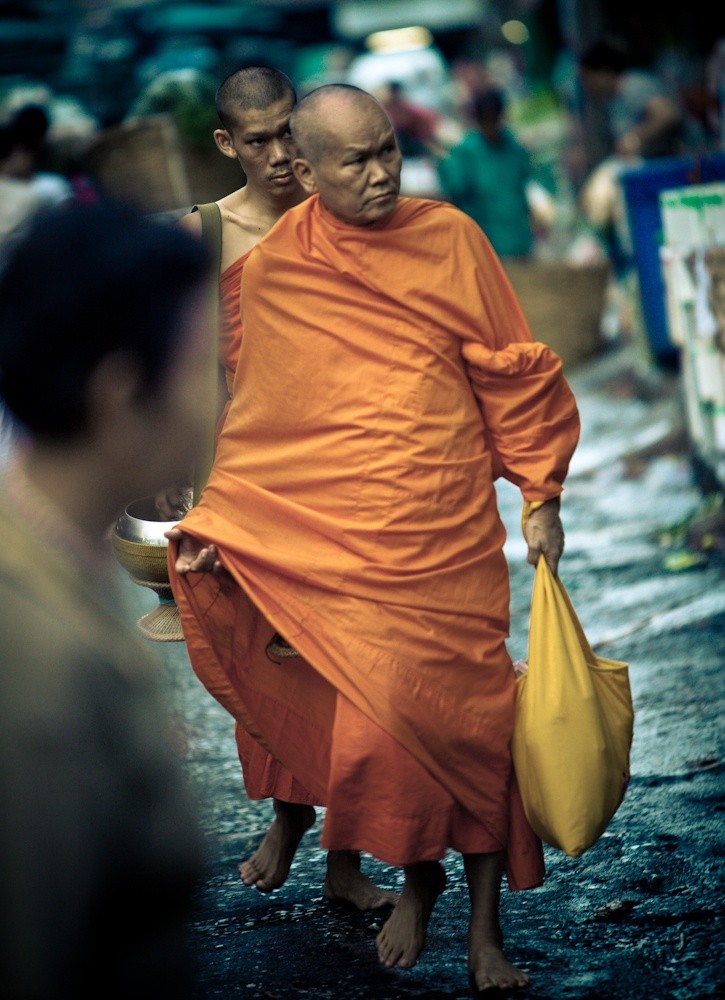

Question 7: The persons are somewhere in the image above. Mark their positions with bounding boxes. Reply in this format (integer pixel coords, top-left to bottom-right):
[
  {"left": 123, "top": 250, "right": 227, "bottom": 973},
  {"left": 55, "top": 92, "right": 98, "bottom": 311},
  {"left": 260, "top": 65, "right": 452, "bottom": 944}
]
[
  {"left": 154, "top": 66, "right": 582, "bottom": 993},
  {"left": 0, "top": 34, "right": 221, "bottom": 1000},
  {"left": 382, "top": 43, "right": 725, "bottom": 261}
]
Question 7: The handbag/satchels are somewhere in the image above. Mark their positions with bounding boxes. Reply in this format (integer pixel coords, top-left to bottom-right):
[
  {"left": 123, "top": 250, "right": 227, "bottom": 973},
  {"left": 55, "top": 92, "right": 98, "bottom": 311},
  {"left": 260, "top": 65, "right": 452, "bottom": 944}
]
[{"left": 510, "top": 549, "right": 635, "bottom": 858}]
